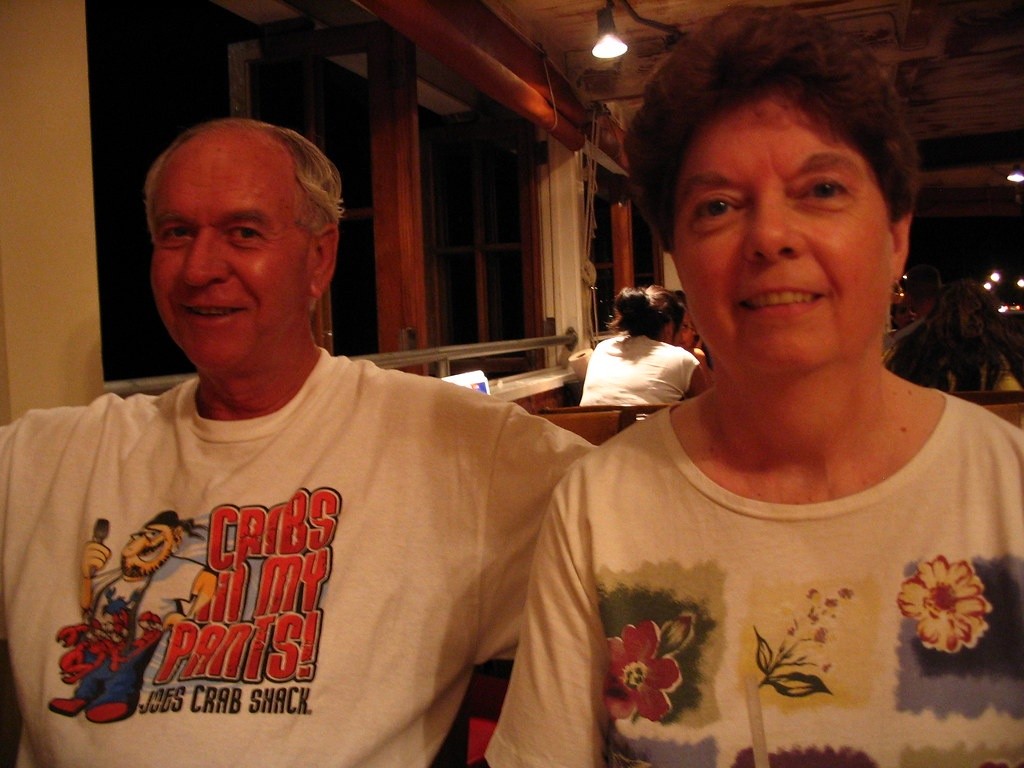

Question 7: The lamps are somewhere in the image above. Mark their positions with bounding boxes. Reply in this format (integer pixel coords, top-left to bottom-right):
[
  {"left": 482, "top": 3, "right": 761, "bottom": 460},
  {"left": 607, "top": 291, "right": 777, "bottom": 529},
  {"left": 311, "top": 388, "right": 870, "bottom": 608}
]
[{"left": 592, "top": 7, "right": 627, "bottom": 58}]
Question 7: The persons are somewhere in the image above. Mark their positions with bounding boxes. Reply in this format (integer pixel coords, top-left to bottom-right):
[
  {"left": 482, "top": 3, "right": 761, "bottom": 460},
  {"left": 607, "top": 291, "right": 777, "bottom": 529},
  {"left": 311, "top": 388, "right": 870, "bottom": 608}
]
[
  {"left": 578, "top": 284, "right": 712, "bottom": 407},
  {"left": 2, "top": 119, "right": 598, "bottom": 768},
  {"left": 885, "top": 265, "right": 1024, "bottom": 392},
  {"left": 484, "top": 6, "right": 1024, "bottom": 768}
]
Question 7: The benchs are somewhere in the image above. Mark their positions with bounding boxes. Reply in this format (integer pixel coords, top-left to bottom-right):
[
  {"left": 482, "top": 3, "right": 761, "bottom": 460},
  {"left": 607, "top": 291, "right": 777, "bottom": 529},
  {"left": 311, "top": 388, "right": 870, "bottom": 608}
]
[{"left": 532, "top": 391, "right": 1024, "bottom": 447}]
